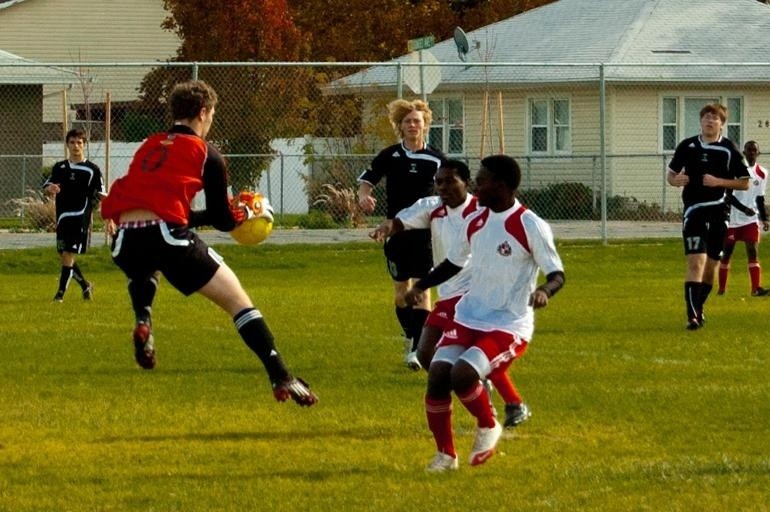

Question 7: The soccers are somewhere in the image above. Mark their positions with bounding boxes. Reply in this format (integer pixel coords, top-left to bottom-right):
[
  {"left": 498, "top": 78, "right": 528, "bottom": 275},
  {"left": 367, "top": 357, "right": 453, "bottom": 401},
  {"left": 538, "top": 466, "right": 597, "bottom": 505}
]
[{"left": 230, "top": 193, "right": 273, "bottom": 246}]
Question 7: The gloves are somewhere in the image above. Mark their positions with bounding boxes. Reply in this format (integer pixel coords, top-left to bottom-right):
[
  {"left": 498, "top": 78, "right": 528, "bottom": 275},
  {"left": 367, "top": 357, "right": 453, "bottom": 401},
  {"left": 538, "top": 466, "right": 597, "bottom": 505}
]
[{"left": 241, "top": 197, "right": 277, "bottom": 226}]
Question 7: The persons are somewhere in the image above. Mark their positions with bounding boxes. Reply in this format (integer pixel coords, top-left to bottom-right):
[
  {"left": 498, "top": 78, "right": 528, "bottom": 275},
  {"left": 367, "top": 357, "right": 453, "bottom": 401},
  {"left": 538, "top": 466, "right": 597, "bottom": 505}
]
[
  {"left": 406, "top": 154, "right": 565, "bottom": 471},
  {"left": 100, "top": 79, "right": 320, "bottom": 406},
  {"left": 42, "top": 127, "right": 108, "bottom": 303},
  {"left": 713, "top": 141, "right": 769, "bottom": 297},
  {"left": 668, "top": 103, "right": 750, "bottom": 328},
  {"left": 357, "top": 101, "right": 445, "bottom": 367},
  {"left": 369, "top": 159, "right": 530, "bottom": 427}
]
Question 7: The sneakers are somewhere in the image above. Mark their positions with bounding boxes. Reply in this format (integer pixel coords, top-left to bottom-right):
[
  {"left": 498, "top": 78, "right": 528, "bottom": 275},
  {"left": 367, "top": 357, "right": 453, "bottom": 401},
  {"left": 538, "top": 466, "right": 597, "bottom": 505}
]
[
  {"left": 503, "top": 399, "right": 532, "bottom": 428},
  {"left": 404, "top": 348, "right": 424, "bottom": 372},
  {"left": 429, "top": 447, "right": 460, "bottom": 474},
  {"left": 50, "top": 289, "right": 65, "bottom": 305},
  {"left": 685, "top": 308, "right": 707, "bottom": 330},
  {"left": 265, "top": 375, "right": 320, "bottom": 408},
  {"left": 130, "top": 323, "right": 159, "bottom": 372},
  {"left": 751, "top": 285, "right": 769, "bottom": 298},
  {"left": 79, "top": 279, "right": 93, "bottom": 302},
  {"left": 466, "top": 415, "right": 503, "bottom": 469}
]
